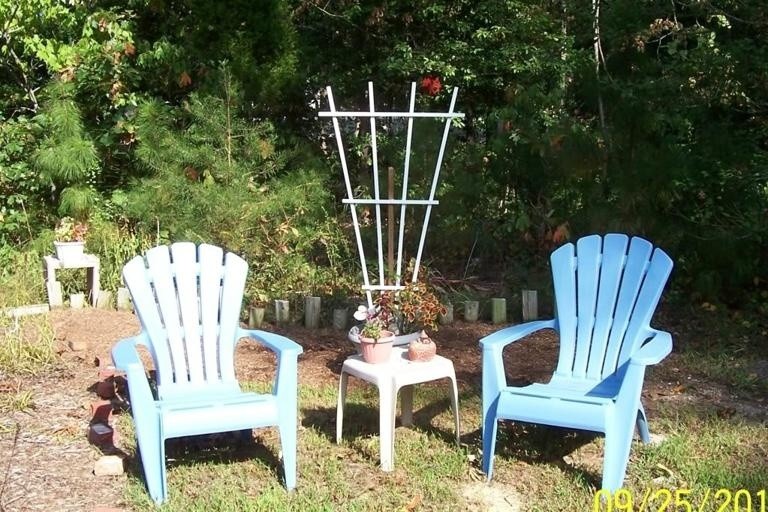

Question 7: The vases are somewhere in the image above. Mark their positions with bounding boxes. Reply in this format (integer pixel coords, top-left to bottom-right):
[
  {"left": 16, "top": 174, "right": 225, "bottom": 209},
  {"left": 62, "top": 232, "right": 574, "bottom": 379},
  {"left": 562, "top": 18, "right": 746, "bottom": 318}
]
[{"left": 347, "top": 323, "right": 423, "bottom": 365}]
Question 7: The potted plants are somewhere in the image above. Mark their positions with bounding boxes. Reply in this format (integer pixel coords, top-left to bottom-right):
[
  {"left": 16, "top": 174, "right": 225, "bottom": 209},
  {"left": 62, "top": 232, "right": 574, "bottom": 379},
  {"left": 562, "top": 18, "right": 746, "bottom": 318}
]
[{"left": 54, "top": 216, "right": 87, "bottom": 259}]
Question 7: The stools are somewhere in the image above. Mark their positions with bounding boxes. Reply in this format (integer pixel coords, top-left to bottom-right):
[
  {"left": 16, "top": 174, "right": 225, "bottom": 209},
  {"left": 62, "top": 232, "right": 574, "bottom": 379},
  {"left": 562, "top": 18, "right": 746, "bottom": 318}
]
[
  {"left": 336, "top": 347, "right": 461, "bottom": 472},
  {"left": 43, "top": 253, "right": 101, "bottom": 307}
]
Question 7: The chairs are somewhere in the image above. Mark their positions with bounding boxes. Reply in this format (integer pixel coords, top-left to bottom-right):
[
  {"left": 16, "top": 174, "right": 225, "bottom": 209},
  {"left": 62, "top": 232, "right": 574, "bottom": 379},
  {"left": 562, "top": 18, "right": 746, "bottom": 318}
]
[
  {"left": 478, "top": 233, "right": 675, "bottom": 504},
  {"left": 111, "top": 241, "right": 304, "bottom": 503}
]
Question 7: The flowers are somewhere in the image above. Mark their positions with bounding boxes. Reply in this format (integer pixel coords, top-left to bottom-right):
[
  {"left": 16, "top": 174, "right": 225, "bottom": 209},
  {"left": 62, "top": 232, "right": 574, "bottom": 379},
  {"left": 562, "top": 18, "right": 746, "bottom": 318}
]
[{"left": 352, "top": 278, "right": 447, "bottom": 339}]
